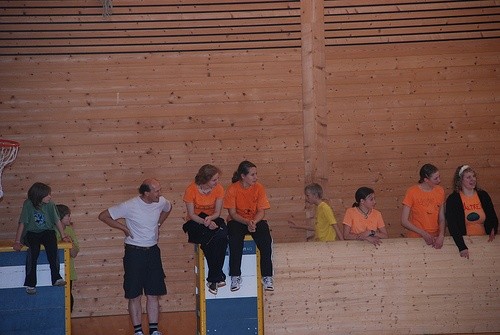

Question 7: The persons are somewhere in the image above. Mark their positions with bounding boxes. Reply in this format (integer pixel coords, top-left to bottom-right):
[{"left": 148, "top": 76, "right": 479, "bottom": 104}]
[
  {"left": 287, "top": 183, "right": 345, "bottom": 241},
  {"left": 401, "top": 163, "right": 446, "bottom": 249},
  {"left": 222, "top": 160, "right": 276, "bottom": 294},
  {"left": 182, "top": 163, "right": 229, "bottom": 295},
  {"left": 54, "top": 204, "right": 80, "bottom": 313},
  {"left": 12, "top": 182, "right": 74, "bottom": 295},
  {"left": 342, "top": 187, "right": 388, "bottom": 247},
  {"left": 444, "top": 164, "right": 499, "bottom": 260},
  {"left": 99, "top": 178, "right": 173, "bottom": 335}
]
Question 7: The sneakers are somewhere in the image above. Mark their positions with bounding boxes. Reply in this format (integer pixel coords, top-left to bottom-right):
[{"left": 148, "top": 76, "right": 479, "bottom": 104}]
[
  {"left": 150, "top": 331, "right": 159, "bottom": 335},
  {"left": 231, "top": 275, "right": 242, "bottom": 291},
  {"left": 134, "top": 331, "right": 144, "bottom": 335},
  {"left": 208, "top": 288, "right": 217, "bottom": 294},
  {"left": 54, "top": 278, "right": 66, "bottom": 286},
  {"left": 26, "top": 286, "right": 35, "bottom": 295},
  {"left": 207, "top": 281, "right": 226, "bottom": 288},
  {"left": 261, "top": 276, "right": 274, "bottom": 290}
]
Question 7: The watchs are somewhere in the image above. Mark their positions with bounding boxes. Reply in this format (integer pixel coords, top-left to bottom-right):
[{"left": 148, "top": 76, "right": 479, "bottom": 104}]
[
  {"left": 369, "top": 230, "right": 375, "bottom": 236},
  {"left": 62, "top": 236, "right": 67, "bottom": 240}
]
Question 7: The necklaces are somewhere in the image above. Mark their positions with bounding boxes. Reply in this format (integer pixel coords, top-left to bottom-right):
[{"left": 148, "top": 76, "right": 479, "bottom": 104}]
[
  {"left": 358, "top": 206, "right": 369, "bottom": 219},
  {"left": 200, "top": 185, "right": 212, "bottom": 196}
]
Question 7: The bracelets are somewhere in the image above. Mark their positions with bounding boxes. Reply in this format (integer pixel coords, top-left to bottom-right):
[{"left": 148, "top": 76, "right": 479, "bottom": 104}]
[
  {"left": 69, "top": 240, "right": 74, "bottom": 243},
  {"left": 158, "top": 222, "right": 162, "bottom": 227},
  {"left": 15, "top": 240, "right": 21, "bottom": 243}
]
